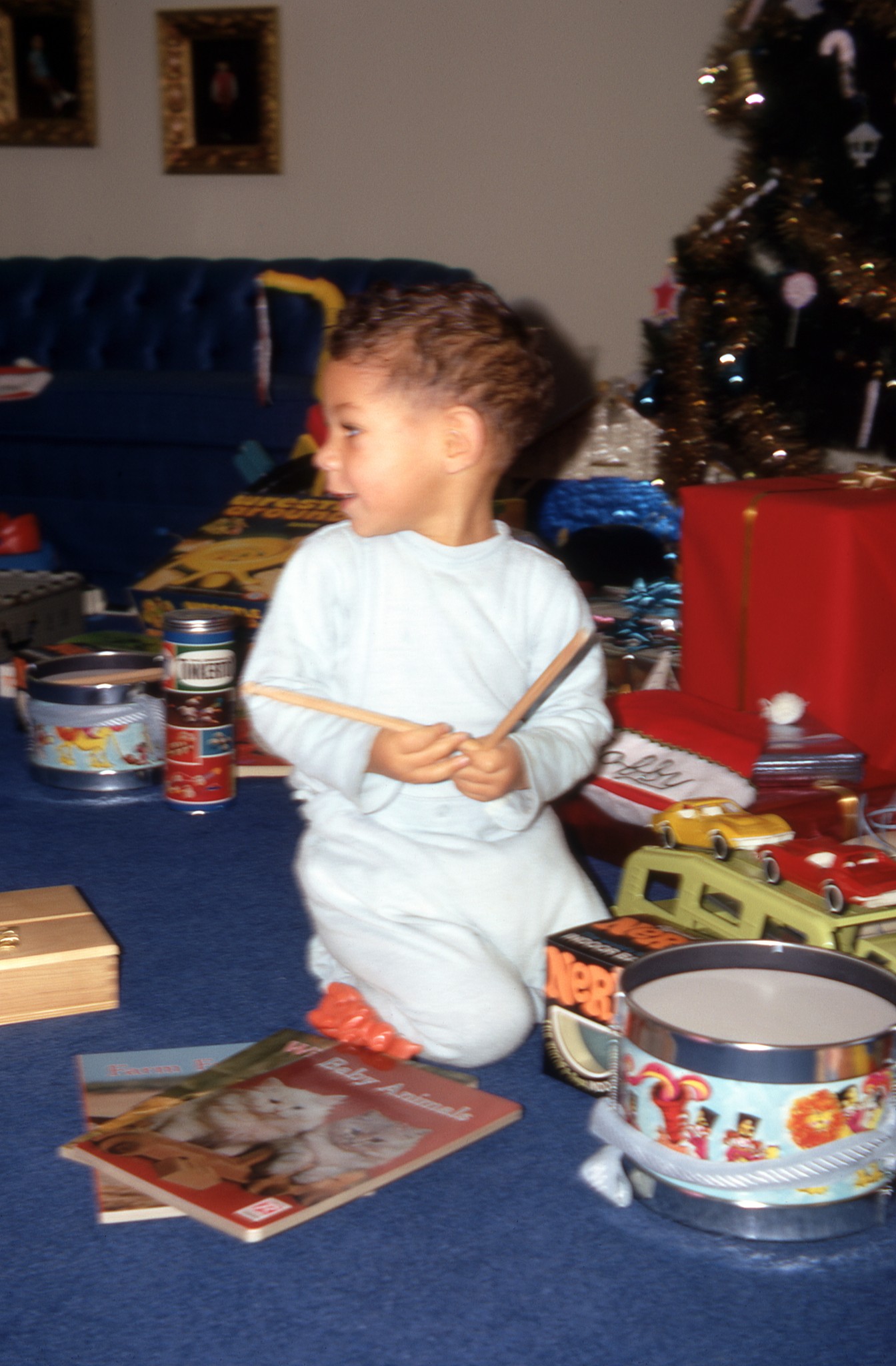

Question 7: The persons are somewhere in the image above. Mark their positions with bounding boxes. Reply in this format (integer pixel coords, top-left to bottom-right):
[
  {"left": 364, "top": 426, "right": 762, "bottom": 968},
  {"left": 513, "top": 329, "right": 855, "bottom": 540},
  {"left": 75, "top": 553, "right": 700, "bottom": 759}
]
[{"left": 244, "top": 272, "right": 627, "bottom": 1066}]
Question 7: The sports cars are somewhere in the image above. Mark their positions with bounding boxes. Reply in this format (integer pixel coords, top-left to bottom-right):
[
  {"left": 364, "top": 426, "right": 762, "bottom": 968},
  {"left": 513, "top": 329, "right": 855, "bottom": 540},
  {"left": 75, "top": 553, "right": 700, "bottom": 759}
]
[{"left": 651, "top": 797, "right": 896, "bottom": 916}]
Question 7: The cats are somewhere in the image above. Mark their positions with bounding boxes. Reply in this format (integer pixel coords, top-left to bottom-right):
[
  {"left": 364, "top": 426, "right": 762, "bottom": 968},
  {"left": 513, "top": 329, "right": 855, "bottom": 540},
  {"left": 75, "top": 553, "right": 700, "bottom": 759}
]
[
  {"left": 137, "top": 1076, "right": 351, "bottom": 1157},
  {"left": 254, "top": 1107, "right": 434, "bottom": 1186}
]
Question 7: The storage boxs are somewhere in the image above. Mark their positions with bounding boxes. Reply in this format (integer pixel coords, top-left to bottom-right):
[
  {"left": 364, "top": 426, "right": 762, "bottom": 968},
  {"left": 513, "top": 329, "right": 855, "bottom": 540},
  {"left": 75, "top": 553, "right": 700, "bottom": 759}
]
[
  {"left": 132, "top": 493, "right": 355, "bottom": 647},
  {"left": 0, "top": 884, "right": 126, "bottom": 1028},
  {"left": 537, "top": 911, "right": 727, "bottom": 1101},
  {"left": 673, "top": 462, "right": 896, "bottom": 798}
]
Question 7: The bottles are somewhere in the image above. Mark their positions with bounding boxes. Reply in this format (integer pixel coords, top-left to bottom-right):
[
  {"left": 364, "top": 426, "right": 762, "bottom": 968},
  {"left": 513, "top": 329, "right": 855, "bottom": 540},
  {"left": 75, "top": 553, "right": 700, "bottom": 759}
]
[{"left": 163, "top": 608, "right": 239, "bottom": 808}]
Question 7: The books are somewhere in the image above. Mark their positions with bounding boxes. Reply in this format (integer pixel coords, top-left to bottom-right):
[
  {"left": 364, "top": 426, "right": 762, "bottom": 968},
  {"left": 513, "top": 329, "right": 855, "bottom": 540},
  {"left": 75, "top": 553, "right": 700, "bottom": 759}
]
[
  {"left": 0, "top": 884, "right": 121, "bottom": 1027},
  {"left": 51, "top": 1023, "right": 526, "bottom": 1245}
]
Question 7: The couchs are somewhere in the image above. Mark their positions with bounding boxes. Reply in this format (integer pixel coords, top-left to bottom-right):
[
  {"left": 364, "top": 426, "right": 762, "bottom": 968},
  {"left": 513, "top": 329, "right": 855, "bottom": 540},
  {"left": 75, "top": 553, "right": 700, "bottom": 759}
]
[{"left": 0, "top": 253, "right": 488, "bottom": 609}]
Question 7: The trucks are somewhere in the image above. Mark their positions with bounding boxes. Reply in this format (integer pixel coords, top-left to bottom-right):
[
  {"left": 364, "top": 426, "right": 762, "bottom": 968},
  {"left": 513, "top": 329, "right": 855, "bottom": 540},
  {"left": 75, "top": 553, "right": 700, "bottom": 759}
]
[{"left": 611, "top": 848, "right": 896, "bottom": 982}]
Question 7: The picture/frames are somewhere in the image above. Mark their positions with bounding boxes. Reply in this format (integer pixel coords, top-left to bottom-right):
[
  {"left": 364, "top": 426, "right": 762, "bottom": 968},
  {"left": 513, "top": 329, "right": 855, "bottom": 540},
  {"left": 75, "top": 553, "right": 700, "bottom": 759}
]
[
  {"left": 148, "top": 8, "right": 289, "bottom": 179},
  {"left": 0, "top": 1, "right": 112, "bottom": 154}
]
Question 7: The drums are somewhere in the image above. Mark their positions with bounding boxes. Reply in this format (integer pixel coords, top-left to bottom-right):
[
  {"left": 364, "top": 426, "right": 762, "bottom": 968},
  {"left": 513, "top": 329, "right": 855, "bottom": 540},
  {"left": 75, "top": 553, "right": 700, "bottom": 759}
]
[
  {"left": 591, "top": 935, "right": 895, "bottom": 1242},
  {"left": 21, "top": 652, "right": 175, "bottom": 794}
]
